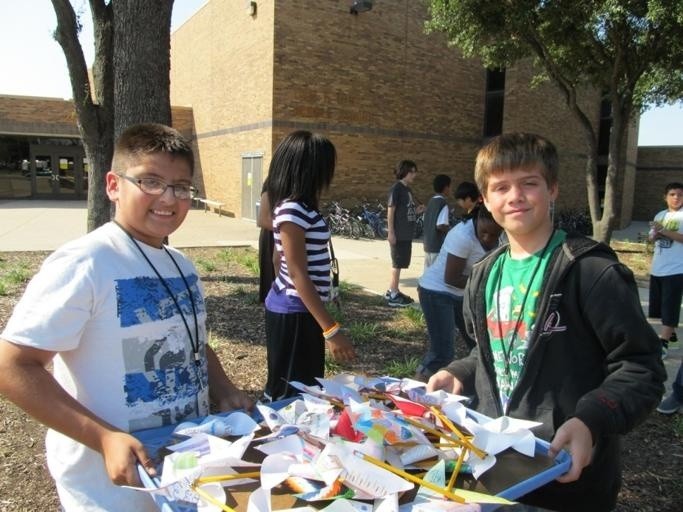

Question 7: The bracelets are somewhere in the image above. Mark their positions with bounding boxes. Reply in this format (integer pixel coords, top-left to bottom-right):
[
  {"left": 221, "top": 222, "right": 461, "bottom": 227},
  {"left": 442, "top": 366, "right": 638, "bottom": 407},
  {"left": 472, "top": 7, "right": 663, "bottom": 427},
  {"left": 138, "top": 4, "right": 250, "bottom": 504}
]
[{"left": 321, "top": 322, "right": 341, "bottom": 340}]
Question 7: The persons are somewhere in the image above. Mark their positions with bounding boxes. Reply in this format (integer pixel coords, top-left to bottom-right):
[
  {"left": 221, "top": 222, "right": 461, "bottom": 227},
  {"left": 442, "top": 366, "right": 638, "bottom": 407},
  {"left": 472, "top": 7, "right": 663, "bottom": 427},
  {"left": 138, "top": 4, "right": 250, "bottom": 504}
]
[
  {"left": 416, "top": 204, "right": 506, "bottom": 383},
  {"left": 648, "top": 183, "right": 683, "bottom": 359},
  {"left": 257, "top": 180, "right": 278, "bottom": 303},
  {"left": 436, "top": 183, "right": 480, "bottom": 253},
  {"left": 656, "top": 361, "right": 683, "bottom": 415},
  {"left": 264, "top": 131, "right": 357, "bottom": 403},
  {"left": 427, "top": 133, "right": 667, "bottom": 511},
  {"left": 1, "top": 122, "right": 253, "bottom": 511},
  {"left": 386, "top": 160, "right": 424, "bottom": 307},
  {"left": 423, "top": 174, "right": 452, "bottom": 275}
]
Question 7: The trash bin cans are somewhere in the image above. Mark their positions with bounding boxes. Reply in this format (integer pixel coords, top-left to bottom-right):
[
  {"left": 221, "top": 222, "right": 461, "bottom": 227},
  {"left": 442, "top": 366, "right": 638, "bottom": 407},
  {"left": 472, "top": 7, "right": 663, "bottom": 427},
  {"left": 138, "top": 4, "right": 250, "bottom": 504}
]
[{"left": 256, "top": 200, "right": 261, "bottom": 227}]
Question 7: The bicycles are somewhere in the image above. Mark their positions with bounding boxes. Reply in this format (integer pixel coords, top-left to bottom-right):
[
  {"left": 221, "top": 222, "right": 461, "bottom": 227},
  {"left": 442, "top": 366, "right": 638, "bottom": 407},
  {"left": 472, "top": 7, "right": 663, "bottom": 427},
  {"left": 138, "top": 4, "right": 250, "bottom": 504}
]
[
  {"left": 551, "top": 208, "right": 593, "bottom": 236},
  {"left": 323, "top": 200, "right": 463, "bottom": 241}
]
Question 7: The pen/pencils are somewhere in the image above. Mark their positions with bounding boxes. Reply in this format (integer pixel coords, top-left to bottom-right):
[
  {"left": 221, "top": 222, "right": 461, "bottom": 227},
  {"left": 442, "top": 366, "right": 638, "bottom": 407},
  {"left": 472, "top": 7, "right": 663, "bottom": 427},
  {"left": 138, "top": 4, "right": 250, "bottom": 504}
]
[
  {"left": 191, "top": 471, "right": 260, "bottom": 512},
  {"left": 318, "top": 392, "right": 490, "bottom": 504}
]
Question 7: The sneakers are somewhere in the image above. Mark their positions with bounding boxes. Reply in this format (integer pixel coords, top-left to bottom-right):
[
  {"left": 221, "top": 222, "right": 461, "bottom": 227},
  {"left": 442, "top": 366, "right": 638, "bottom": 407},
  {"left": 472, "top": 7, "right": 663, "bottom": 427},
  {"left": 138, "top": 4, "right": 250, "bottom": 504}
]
[
  {"left": 660, "top": 339, "right": 679, "bottom": 359},
  {"left": 385, "top": 290, "right": 414, "bottom": 307},
  {"left": 658, "top": 392, "right": 681, "bottom": 413}
]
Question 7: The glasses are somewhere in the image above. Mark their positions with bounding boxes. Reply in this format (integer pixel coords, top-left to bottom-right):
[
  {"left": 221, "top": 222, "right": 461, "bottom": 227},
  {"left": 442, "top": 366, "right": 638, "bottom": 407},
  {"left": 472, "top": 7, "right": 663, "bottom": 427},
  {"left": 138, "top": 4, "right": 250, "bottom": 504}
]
[{"left": 119, "top": 175, "right": 198, "bottom": 200}]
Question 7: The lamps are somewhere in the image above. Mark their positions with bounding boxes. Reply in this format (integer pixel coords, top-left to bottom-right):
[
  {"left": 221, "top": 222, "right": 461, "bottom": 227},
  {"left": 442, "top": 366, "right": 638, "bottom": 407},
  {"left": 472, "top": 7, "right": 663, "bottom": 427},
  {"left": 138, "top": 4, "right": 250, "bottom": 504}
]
[
  {"left": 246, "top": 0, "right": 256, "bottom": 15},
  {"left": 350, "top": 0, "right": 372, "bottom": 15}
]
